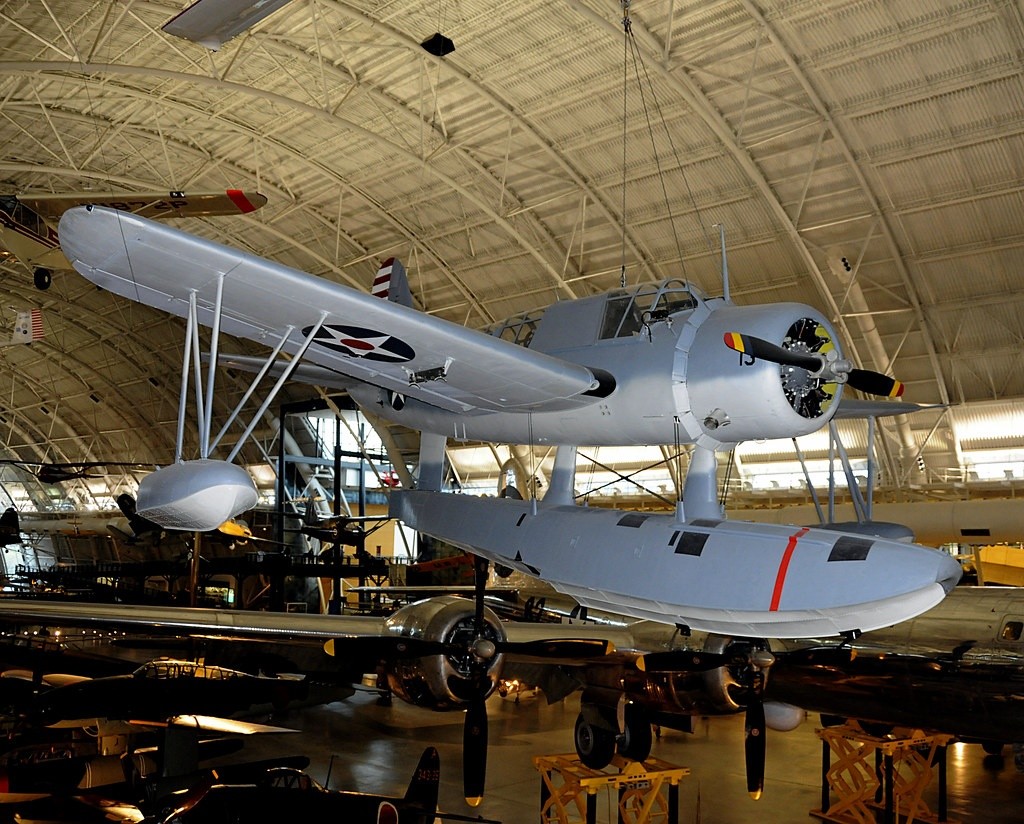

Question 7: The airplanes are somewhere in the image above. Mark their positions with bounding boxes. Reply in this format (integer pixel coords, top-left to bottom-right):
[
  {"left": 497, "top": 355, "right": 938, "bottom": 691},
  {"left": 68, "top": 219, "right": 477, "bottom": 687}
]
[{"left": 1, "top": 179, "right": 1023, "bottom": 824}]
